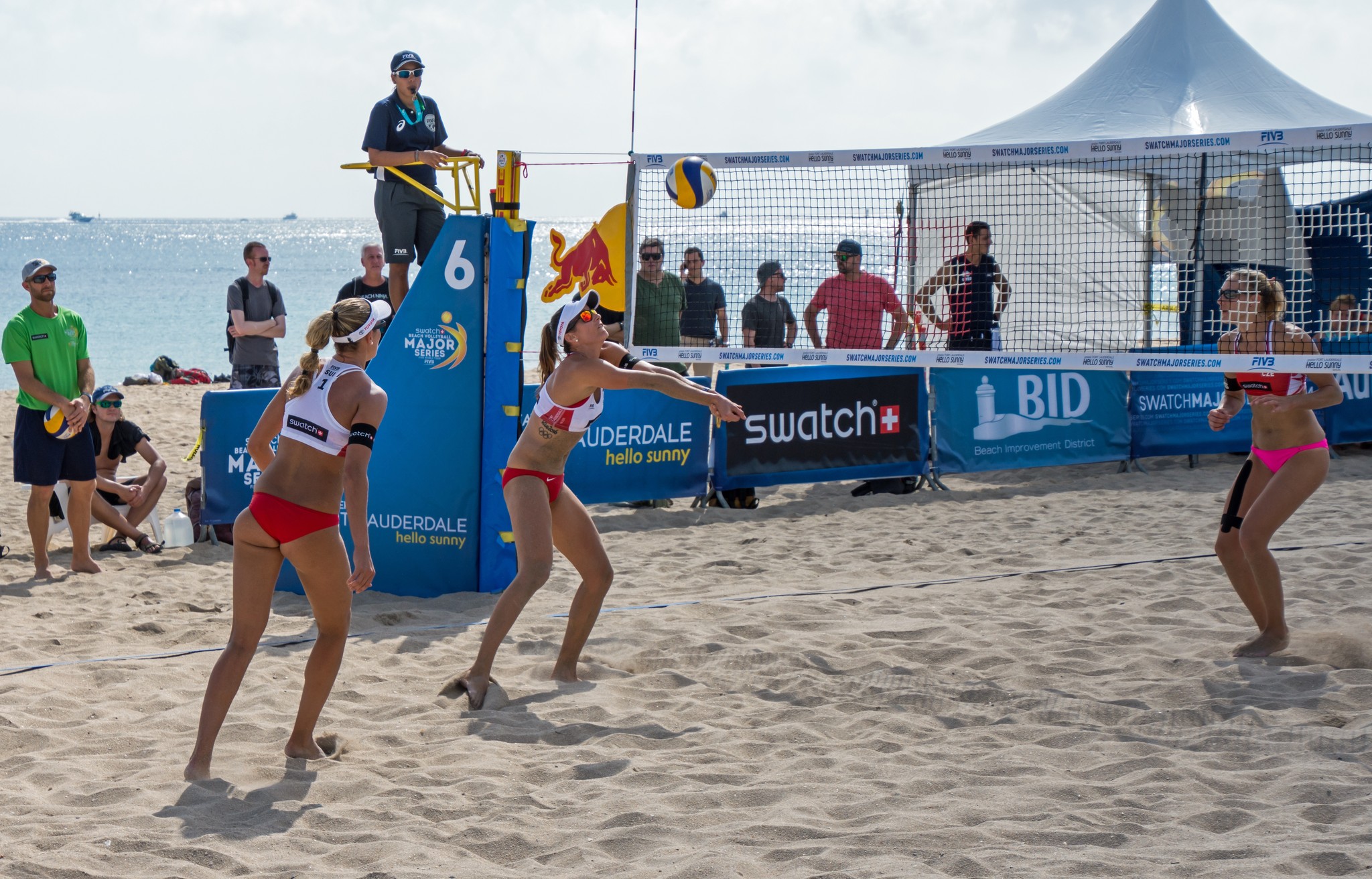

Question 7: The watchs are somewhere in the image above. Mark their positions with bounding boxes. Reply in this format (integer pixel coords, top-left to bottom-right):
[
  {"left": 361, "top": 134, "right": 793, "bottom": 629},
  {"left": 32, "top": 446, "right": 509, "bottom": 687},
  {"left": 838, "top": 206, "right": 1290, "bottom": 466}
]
[
  {"left": 80, "top": 392, "right": 93, "bottom": 404},
  {"left": 786, "top": 343, "right": 793, "bottom": 348},
  {"left": 723, "top": 342, "right": 729, "bottom": 347},
  {"left": 618, "top": 321, "right": 624, "bottom": 331}
]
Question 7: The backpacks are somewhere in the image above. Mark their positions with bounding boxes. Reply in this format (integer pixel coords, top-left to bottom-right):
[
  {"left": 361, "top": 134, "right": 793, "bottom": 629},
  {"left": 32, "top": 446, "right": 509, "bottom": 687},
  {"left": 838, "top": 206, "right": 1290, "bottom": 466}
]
[
  {"left": 851, "top": 476, "right": 917, "bottom": 496},
  {"left": 709, "top": 479, "right": 760, "bottom": 509},
  {"left": 150, "top": 355, "right": 214, "bottom": 384},
  {"left": 224, "top": 276, "right": 278, "bottom": 362}
]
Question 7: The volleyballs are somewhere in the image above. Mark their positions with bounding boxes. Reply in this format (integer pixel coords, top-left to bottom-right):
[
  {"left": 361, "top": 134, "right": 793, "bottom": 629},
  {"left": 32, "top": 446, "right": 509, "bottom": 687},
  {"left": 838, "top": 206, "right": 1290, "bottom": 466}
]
[
  {"left": 665, "top": 156, "right": 716, "bottom": 208},
  {"left": 44, "top": 405, "right": 80, "bottom": 439}
]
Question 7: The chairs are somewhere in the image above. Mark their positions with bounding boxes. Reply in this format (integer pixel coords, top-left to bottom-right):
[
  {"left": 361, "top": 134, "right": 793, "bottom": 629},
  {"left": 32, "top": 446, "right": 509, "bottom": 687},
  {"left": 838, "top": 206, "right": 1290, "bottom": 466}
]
[{"left": 20, "top": 476, "right": 165, "bottom": 553}]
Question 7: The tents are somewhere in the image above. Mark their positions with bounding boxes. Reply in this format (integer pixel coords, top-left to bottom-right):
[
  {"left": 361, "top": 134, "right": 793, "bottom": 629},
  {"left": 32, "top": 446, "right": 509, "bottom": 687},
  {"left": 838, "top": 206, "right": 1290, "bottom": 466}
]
[{"left": 908, "top": 0, "right": 1372, "bottom": 350}]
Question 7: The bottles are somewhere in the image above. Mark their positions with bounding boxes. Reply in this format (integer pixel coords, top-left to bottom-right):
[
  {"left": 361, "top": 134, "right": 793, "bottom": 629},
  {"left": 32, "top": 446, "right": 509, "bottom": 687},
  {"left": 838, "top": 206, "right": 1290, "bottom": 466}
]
[{"left": 163, "top": 509, "right": 194, "bottom": 548}]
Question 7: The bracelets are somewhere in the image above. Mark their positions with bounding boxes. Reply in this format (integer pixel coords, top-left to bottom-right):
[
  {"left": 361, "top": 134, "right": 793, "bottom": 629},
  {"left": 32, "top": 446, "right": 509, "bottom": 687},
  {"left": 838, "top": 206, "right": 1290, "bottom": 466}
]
[
  {"left": 415, "top": 149, "right": 419, "bottom": 162},
  {"left": 348, "top": 422, "right": 377, "bottom": 452},
  {"left": 619, "top": 353, "right": 641, "bottom": 371},
  {"left": 1360, "top": 313, "right": 1364, "bottom": 320},
  {"left": 461, "top": 149, "right": 472, "bottom": 157},
  {"left": 702, "top": 387, "right": 710, "bottom": 392},
  {"left": 1222, "top": 374, "right": 1243, "bottom": 391}
]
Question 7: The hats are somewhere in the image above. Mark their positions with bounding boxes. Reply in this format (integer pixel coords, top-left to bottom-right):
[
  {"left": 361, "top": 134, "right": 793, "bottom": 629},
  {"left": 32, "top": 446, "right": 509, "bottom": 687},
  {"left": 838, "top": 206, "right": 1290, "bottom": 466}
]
[
  {"left": 827, "top": 239, "right": 862, "bottom": 254},
  {"left": 556, "top": 290, "right": 601, "bottom": 346},
  {"left": 331, "top": 297, "right": 392, "bottom": 343},
  {"left": 390, "top": 50, "right": 425, "bottom": 70},
  {"left": 93, "top": 385, "right": 124, "bottom": 404},
  {"left": 755, "top": 260, "right": 782, "bottom": 292},
  {"left": 22, "top": 258, "right": 57, "bottom": 282}
]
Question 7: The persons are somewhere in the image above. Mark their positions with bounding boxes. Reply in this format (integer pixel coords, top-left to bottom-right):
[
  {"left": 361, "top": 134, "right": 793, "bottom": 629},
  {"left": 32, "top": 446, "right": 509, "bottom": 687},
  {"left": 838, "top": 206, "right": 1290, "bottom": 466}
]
[
  {"left": 677, "top": 246, "right": 732, "bottom": 347},
  {"left": 225, "top": 240, "right": 287, "bottom": 390},
  {"left": 2, "top": 259, "right": 101, "bottom": 579},
  {"left": 632, "top": 238, "right": 687, "bottom": 346},
  {"left": 361, "top": 50, "right": 484, "bottom": 315},
  {"left": 335, "top": 243, "right": 395, "bottom": 368},
  {"left": 185, "top": 297, "right": 392, "bottom": 784},
  {"left": 59, "top": 385, "right": 168, "bottom": 554},
  {"left": 804, "top": 239, "right": 907, "bottom": 348},
  {"left": 572, "top": 290, "right": 624, "bottom": 345},
  {"left": 1206, "top": 267, "right": 1343, "bottom": 657},
  {"left": 1312, "top": 293, "right": 1372, "bottom": 340},
  {"left": 459, "top": 289, "right": 747, "bottom": 711},
  {"left": 916, "top": 221, "right": 1012, "bottom": 351},
  {"left": 741, "top": 260, "right": 798, "bottom": 370}
]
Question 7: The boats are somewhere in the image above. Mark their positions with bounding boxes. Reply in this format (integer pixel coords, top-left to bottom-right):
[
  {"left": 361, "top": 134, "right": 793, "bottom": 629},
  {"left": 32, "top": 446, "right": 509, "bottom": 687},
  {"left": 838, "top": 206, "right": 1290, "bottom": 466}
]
[
  {"left": 284, "top": 213, "right": 297, "bottom": 219},
  {"left": 69, "top": 212, "right": 94, "bottom": 222}
]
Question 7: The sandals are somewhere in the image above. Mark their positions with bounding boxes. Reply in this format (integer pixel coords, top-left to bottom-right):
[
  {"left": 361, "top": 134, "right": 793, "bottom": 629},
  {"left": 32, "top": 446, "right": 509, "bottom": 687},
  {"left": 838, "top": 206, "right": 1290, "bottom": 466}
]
[
  {"left": 135, "top": 534, "right": 163, "bottom": 553},
  {"left": 99, "top": 537, "right": 133, "bottom": 552}
]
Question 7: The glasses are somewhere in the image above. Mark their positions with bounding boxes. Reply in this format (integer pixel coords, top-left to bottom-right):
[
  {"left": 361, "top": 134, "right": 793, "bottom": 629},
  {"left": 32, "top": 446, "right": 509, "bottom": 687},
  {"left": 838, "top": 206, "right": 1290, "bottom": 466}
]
[
  {"left": 95, "top": 400, "right": 122, "bottom": 408},
  {"left": 1219, "top": 288, "right": 1260, "bottom": 299},
  {"left": 566, "top": 309, "right": 598, "bottom": 332},
  {"left": 772, "top": 272, "right": 784, "bottom": 277},
  {"left": 640, "top": 253, "right": 662, "bottom": 261},
  {"left": 254, "top": 256, "right": 271, "bottom": 261},
  {"left": 392, "top": 69, "right": 424, "bottom": 78},
  {"left": 834, "top": 254, "right": 858, "bottom": 261},
  {"left": 373, "top": 320, "right": 386, "bottom": 333},
  {"left": 27, "top": 273, "right": 56, "bottom": 284}
]
